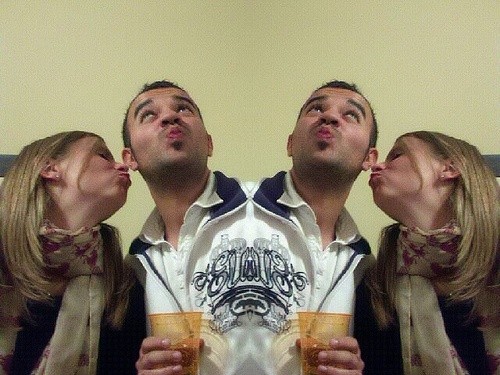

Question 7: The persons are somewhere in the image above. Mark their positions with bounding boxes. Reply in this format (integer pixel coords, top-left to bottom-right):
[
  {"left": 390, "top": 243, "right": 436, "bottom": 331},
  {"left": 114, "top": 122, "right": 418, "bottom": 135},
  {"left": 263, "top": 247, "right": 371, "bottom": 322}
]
[
  {"left": 353, "top": 131, "right": 500, "bottom": 375},
  {"left": 0, "top": 131, "right": 147, "bottom": 375},
  {"left": 121, "top": 80, "right": 250, "bottom": 375},
  {"left": 250, "top": 81, "right": 378, "bottom": 375}
]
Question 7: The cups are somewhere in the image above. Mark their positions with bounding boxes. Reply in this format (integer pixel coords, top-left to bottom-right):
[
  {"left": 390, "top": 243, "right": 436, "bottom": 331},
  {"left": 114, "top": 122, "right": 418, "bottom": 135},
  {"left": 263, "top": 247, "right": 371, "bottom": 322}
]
[
  {"left": 296, "top": 312, "right": 352, "bottom": 375},
  {"left": 147, "top": 311, "right": 203, "bottom": 375}
]
